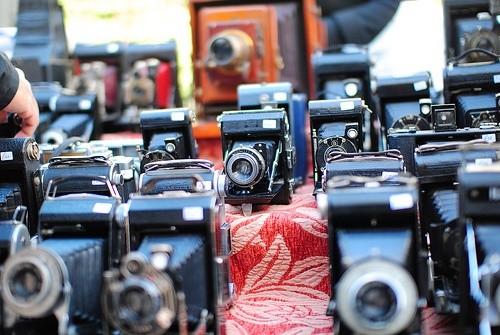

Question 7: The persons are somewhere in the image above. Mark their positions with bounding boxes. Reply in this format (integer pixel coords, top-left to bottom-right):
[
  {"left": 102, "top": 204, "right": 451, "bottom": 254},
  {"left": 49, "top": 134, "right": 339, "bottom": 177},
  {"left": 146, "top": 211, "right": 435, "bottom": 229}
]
[
  {"left": 0, "top": 47, "right": 39, "bottom": 138},
  {"left": 316, "top": 0, "right": 400, "bottom": 46}
]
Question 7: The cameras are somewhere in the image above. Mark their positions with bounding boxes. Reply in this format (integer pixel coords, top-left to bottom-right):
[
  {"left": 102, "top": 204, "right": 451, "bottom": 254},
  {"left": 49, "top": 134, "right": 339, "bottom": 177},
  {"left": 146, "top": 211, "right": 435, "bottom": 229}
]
[
  {"left": 0, "top": 1, "right": 181, "bottom": 114},
  {"left": 238, "top": 78, "right": 308, "bottom": 184},
  {"left": 307, "top": 47, "right": 500, "bottom": 335},
  {"left": 0, "top": 83, "right": 233, "bottom": 335},
  {"left": 218, "top": 109, "right": 294, "bottom": 205},
  {"left": 190, "top": 0, "right": 324, "bottom": 115},
  {"left": 442, "top": 0, "right": 500, "bottom": 68}
]
